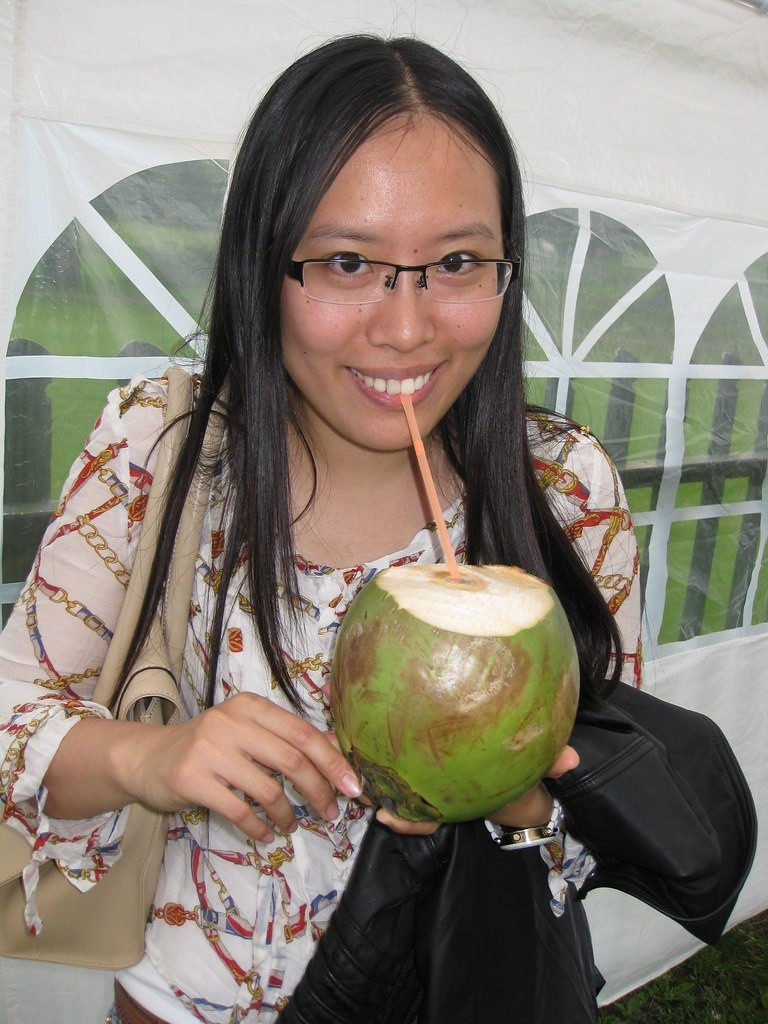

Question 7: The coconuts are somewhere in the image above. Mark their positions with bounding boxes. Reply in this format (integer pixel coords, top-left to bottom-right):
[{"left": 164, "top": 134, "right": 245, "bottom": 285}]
[{"left": 332, "top": 563, "right": 582, "bottom": 825}]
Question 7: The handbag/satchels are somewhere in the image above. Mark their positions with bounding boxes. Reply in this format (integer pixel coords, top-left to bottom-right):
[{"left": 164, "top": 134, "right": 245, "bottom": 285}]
[{"left": 0, "top": 366, "right": 232, "bottom": 969}]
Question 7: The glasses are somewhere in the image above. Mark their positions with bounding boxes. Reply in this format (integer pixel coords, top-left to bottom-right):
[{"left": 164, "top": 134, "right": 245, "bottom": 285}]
[{"left": 282, "top": 238, "right": 522, "bottom": 304}]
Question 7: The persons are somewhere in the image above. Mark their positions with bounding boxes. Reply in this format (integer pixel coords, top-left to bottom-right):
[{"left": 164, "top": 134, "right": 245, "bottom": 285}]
[{"left": 0, "top": 35, "right": 647, "bottom": 1024}]
